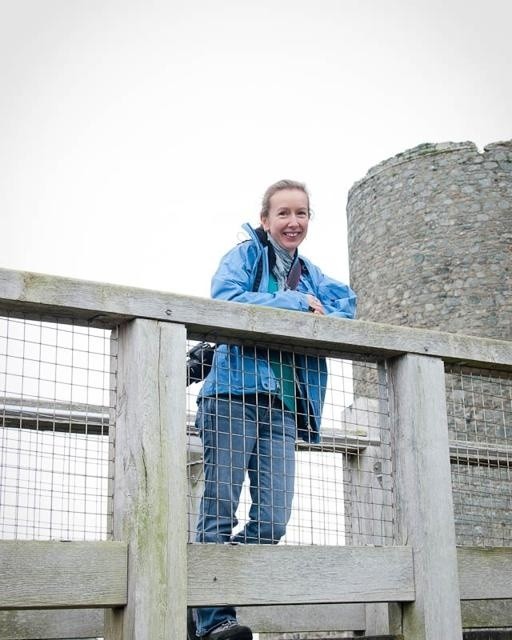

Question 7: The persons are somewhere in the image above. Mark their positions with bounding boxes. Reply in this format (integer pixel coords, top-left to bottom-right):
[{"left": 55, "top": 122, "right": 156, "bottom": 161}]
[{"left": 189, "top": 178, "right": 358, "bottom": 640}]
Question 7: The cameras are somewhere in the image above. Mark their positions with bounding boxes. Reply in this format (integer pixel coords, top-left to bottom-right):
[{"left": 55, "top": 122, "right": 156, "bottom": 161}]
[{"left": 186, "top": 342, "right": 214, "bottom": 387}]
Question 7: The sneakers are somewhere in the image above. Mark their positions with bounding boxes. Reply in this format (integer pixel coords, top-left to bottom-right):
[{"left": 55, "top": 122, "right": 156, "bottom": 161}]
[{"left": 203, "top": 618, "right": 253, "bottom": 640}]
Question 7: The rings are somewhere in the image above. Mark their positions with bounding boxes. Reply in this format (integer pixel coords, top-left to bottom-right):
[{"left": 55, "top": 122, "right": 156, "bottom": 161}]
[{"left": 313, "top": 297, "right": 319, "bottom": 302}]
